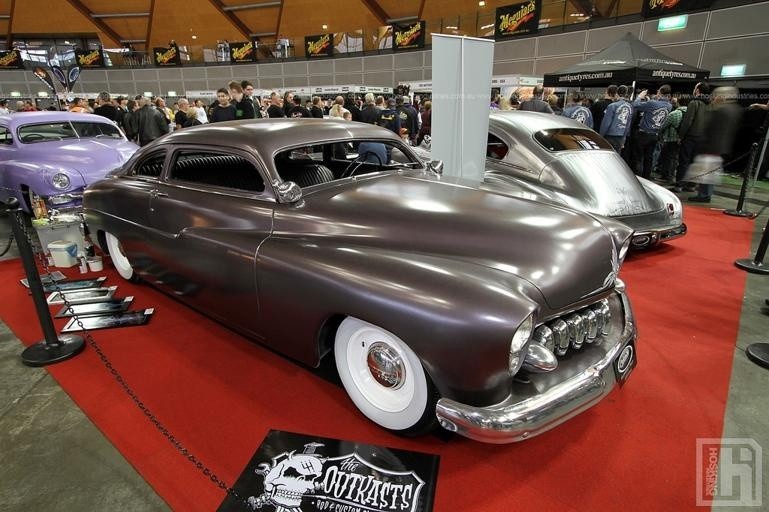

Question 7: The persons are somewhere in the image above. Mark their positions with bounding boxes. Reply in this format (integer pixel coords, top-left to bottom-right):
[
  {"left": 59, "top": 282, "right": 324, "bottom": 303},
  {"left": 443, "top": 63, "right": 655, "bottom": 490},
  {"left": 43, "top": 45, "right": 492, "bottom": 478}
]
[{"left": 1, "top": 80, "right": 769, "bottom": 203}]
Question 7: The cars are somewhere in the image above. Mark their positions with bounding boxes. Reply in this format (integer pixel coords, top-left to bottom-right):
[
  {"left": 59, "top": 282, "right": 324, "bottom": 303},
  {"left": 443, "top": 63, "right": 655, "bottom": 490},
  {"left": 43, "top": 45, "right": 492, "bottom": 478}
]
[
  {"left": 1, "top": 109, "right": 139, "bottom": 215},
  {"left": 80, "top": 113, "right": 638, "bottom": 439},
  {"left": 416, "top": 108, "right": 687, "bottom": 255}
]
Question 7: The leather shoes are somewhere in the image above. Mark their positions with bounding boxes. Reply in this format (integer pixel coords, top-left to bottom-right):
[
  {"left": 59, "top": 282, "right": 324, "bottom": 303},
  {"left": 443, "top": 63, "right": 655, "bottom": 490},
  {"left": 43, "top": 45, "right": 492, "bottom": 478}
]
[{"left": 655, "top": 175, "right": 710, "bottom": 203}]
